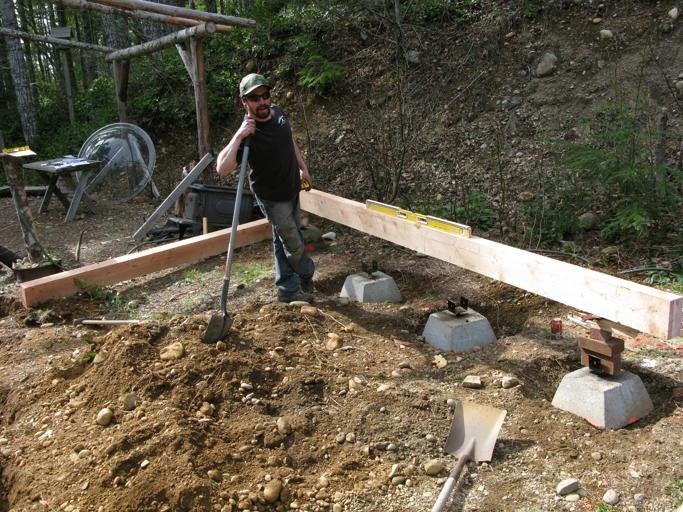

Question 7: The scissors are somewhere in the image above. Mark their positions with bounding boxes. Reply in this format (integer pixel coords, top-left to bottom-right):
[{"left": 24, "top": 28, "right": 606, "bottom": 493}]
[
  {"left": 429, "top": 400, "right": 506, "bottom": 511},
  {"left": 203, "top": 138, "right": 249, "bottom": 342}
]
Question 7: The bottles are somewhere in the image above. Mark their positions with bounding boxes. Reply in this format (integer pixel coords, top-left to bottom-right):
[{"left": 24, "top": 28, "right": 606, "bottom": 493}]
[{"left": 239, "top": 72, "right": 273, "bottom": 97}]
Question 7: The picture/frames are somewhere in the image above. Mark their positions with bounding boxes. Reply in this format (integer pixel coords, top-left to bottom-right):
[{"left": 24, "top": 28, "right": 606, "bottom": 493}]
[{"left": 23, "top": 155, "right": 103, "bottom": 223}]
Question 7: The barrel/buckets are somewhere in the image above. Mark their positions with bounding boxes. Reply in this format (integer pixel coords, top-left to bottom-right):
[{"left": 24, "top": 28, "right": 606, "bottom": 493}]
[{"left": 247, "top": 92, "right": 270, "bottom": 101}]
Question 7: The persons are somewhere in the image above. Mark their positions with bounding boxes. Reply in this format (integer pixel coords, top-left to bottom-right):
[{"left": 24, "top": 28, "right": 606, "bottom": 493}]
[{"left": 216, "top": 73, "right": 315, "bottom": 303}]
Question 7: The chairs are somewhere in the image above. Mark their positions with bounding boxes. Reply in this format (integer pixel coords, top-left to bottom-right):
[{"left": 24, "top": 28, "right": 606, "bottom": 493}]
[{"left": 277, "top": 271, "right": 314, "bottom": 302}]
